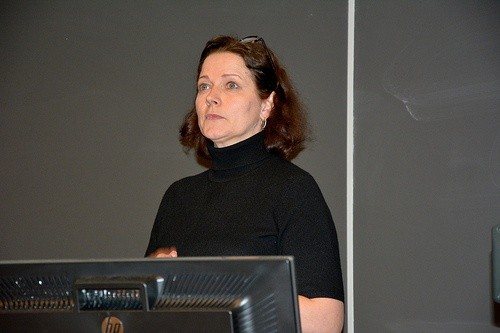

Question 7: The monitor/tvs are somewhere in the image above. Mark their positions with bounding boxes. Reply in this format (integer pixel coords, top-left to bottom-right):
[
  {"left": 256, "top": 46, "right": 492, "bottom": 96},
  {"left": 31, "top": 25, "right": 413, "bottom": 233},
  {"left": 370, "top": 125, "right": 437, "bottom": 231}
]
[{"left": 0, "top": 255, "right": 302, "bottom": 333}]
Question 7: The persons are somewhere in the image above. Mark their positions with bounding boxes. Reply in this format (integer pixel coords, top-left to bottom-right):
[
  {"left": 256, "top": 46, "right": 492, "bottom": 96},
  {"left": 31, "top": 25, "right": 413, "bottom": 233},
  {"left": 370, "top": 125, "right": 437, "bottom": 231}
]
[{"left": 144, "top": 35, "right": 345, "bottom": 333}]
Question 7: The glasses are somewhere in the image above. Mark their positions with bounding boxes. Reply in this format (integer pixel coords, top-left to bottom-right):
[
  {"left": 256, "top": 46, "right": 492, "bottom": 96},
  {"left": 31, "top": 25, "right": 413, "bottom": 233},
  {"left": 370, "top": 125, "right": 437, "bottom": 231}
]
[{"left": 239, "top": 35, "right": 281, "bottom": 89}]
